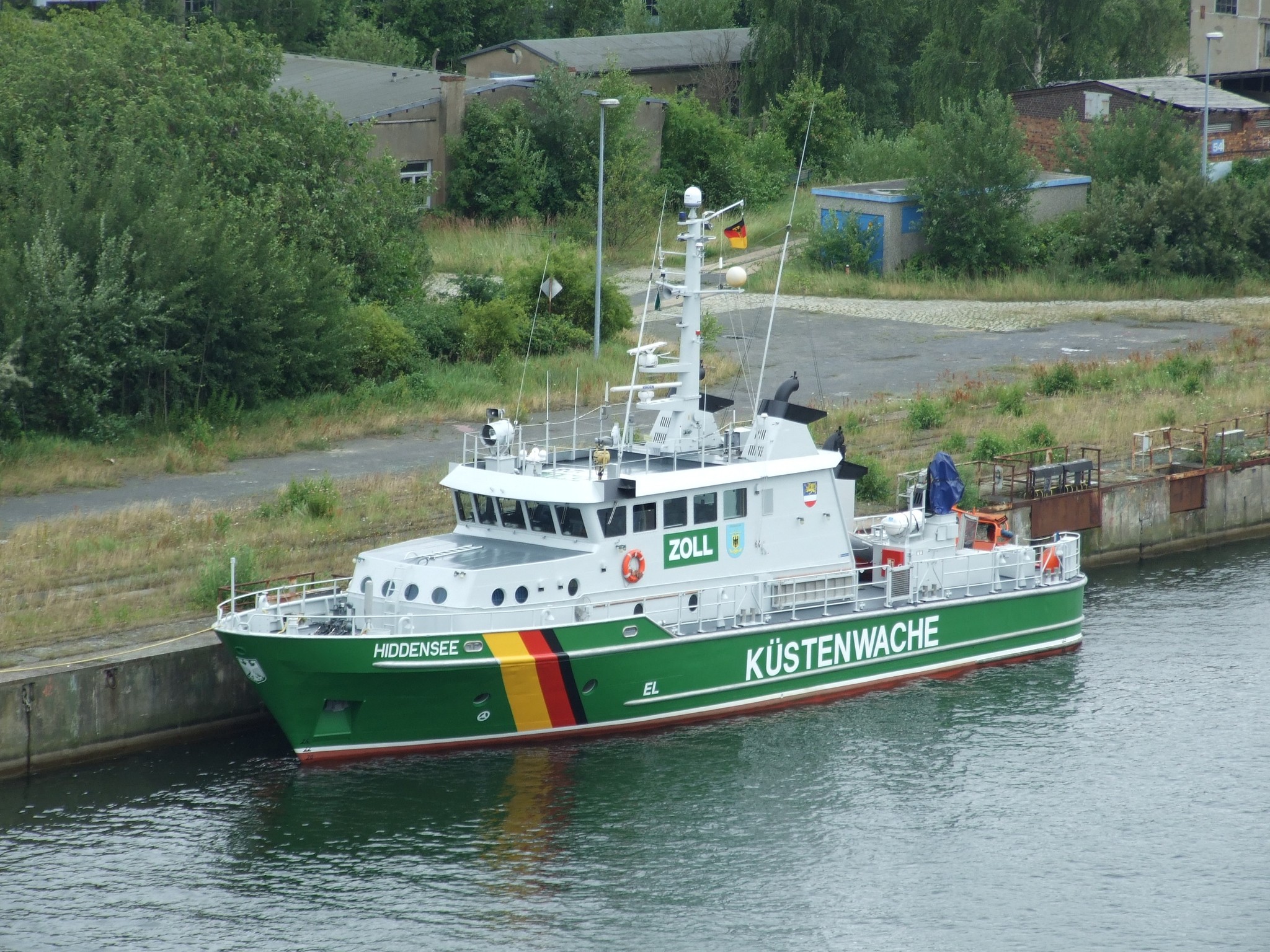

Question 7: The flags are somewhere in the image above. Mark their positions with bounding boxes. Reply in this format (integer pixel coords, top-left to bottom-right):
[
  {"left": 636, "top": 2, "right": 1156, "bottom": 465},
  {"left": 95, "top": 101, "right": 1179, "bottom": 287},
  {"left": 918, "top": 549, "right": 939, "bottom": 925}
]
[
  {"left": 724, "top": 217, "right": 747, "bottom": 249},
  {"left": 654, "top": 291, "right": 662, "bottom": 312}
]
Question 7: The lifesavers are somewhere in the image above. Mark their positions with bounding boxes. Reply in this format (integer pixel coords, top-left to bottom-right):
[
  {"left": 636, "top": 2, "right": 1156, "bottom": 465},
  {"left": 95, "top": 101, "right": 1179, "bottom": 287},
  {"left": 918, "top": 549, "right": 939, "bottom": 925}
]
[{"left": 622, "top": 549, "right": 645, "bottom": 584}]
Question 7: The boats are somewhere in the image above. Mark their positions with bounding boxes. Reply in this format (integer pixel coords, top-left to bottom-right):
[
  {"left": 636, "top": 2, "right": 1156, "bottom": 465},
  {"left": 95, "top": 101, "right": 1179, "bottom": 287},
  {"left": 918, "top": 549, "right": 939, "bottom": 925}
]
[{"left": 212, "top": 186, "right": 1087, "bottom": 769}]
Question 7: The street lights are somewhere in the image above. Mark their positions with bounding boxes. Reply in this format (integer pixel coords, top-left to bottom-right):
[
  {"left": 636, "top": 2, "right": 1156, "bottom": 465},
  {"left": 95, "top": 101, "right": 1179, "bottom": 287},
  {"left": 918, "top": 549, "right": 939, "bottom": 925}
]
[
  {"left": 590, "top": 98, "right": 620, "bottom": 358},
  {"left": 1202, "top": 33, "right": 1225, "bottom": 189}
]
